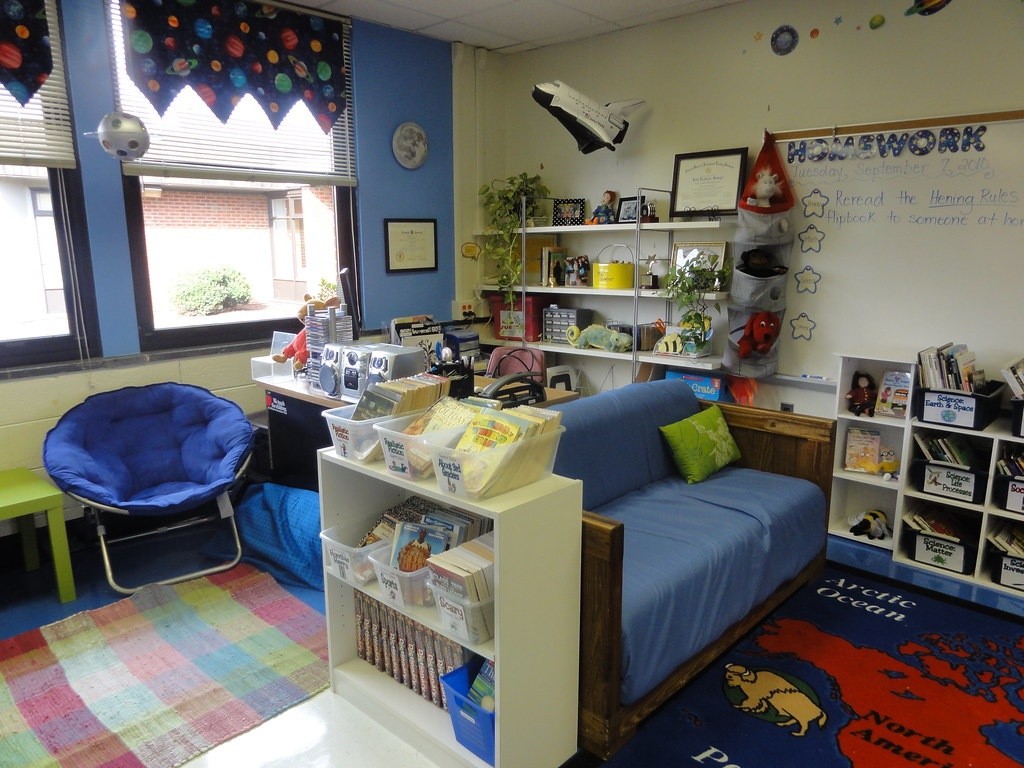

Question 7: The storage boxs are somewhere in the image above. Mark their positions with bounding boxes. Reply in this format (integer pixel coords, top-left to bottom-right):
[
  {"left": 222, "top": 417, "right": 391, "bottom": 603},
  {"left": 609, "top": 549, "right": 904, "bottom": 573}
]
[
  {"left": 1009, "top": 395, "right": 1024, "bottom": 438},
  {"left": 988, "top": 544, "right": 1024, "bottom": 591},
  {"left": 914, "top": 379, "right": 1008, "bottom": 432},
  {"left": 320, "top": 404, "right": 429, "bottom": 465},
  {"left": 906, "top": 526, "right": 980, "bottom": 576},
  {"left": 665, "top": 371, "right": 735, "bottom": 403},
  {"left": 421, "top": 424, "right": 566, "bottom": 502},
  {"left": 996, "top": 469, "right": 1024, "bottom": 515},
  {"left": 440, "top": 654, "right": 495, "bottom": 768},
  {"left": 425, "top": 576, "right": 495, "bottom": 646},
  {"left": 653, "top": 327, "right": 713, "bottom": 358},
  {"left": 319, "top": 517, "right": 394, "bottom": 588},
  {"left": 372, "top": 411, "right": 495, "bottom": 483},
  {"left": 367, "top": 545, "right": 431, "bottom": 613},
  {"left": 914, "top": 453, "right": 992, "bottom": 505},
  {"left": 489, "top": 295, "right": 563, "bottom": 341}
]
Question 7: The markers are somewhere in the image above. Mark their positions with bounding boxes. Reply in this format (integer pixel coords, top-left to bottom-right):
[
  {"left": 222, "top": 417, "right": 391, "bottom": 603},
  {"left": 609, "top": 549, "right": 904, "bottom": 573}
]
[{"left": 801, "top": 374, "right": 829, "bottom": 380}]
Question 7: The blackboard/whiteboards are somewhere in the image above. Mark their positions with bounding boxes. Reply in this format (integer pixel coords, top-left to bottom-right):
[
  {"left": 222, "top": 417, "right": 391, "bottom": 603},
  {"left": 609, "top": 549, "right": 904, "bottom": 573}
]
[{"left": 763, "top": 108, "right": 1024, "bottom": 386}]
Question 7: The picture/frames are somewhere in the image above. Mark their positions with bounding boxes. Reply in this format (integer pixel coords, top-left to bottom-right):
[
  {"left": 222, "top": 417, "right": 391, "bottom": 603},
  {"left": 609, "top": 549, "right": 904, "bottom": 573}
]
[
  {"left": 552, "top": 198, "right": 585, "bottom": 226},
  {"left": 384, "top": 217, "right": 439, "bottom": 274},
  {"left": 669, "top": 146, "right": 749, "bottom": 217},
  {"left": 615, "top": 195, "right": 646, "bottom": 224},
  {"left": 666, "top": 241, "right": 728, "bottom": 289}
]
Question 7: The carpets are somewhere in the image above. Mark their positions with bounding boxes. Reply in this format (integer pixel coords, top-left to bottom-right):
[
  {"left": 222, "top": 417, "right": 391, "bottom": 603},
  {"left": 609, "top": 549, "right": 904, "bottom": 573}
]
[
  {"left": 596, "top": 559, "right": 1024, "bottom": 768},
  {"left": 0, "top": 561, "right": 330, "bottom": 768}
]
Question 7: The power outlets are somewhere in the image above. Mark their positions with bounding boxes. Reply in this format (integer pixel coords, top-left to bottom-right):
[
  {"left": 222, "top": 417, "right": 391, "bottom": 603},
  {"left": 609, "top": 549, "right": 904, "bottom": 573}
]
[{"left": 781, "top": 402, "right": 794, "bottom": 413}]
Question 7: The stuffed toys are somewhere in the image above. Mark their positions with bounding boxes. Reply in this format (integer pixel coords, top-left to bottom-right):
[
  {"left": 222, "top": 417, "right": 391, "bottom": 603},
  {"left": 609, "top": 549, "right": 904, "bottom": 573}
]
[
  {"left": 846, "top": 509, "right": 893, "bottom": 540},
  {"left": 753, "top": 173, "right": 784, "bottom": 208},
  {"left": 740, "top": 249, "right": 788, "bottom": 279},
  {"left": 566, "top": 324, "right": 633, "bottom": 353},
  {"left": 584, "top": 190, "right": 615, "bottom": 225},
  {"left": 878, "top": 445, "right": 901, "bottom": 480},
  {"left": 271, "top": 294, "right": 341, "bottom": 371},
  {"left": 844, "top": 371, "right": 876, "bottom": 417},
  {"left": 736, "top": 311, "right": 780, "bottom": 359}
]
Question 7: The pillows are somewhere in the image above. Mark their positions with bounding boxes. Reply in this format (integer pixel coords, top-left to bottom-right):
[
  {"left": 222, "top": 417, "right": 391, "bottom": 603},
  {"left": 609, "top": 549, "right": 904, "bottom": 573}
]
[{"left": 658, "top": 403, "right": 742, "bottom": 484}]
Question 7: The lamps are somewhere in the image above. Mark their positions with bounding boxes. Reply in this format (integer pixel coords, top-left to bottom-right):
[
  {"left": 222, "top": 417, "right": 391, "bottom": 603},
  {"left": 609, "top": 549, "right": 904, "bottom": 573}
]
[{"left": 144, "top": 185, "right": 162, "bottom": 198}]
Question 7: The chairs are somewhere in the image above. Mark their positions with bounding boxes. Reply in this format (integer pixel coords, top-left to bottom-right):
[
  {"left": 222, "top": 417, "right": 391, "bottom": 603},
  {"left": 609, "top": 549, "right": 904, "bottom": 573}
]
[
  {"left": 484, "top": 347, "right": 547, "bottom": 388},
  {"left": 42, "top": 379, "right": 258, "bottom": 594}
]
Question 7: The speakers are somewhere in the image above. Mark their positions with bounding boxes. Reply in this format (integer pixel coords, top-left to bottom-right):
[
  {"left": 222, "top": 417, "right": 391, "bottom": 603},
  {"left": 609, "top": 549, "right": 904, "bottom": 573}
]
[
  {"left": 368, "top": 346, "right": 426, "bottom": 384},
  {"left": 318, "top": 342, "right": 376, "bottom": 401}
]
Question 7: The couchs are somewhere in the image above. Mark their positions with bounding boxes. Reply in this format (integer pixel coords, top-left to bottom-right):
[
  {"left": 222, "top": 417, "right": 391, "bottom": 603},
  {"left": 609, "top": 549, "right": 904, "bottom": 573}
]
[{"left": 544, "top": 379, "right": 837, "bottom": 761}]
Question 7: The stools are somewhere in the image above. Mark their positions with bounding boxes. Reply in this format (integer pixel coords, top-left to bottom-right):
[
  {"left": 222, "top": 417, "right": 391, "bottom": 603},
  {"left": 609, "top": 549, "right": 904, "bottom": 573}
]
[{"left": 0, "top": 466, "right": 77, "bottom": 605}]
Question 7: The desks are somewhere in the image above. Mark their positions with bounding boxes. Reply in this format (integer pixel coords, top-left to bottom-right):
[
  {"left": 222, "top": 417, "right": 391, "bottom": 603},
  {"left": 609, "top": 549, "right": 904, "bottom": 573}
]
[{"left": 256, "top": 369, "right": 581, "bottom": 493}]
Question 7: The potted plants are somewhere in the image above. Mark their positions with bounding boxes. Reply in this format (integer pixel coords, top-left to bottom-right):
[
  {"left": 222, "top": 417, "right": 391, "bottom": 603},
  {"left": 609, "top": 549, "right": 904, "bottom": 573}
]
[
  {"left": 653, "top": 250, "right": 734, "bottom": 354},
  {"left": 477, "top": 163, "right": 554, "bottom": 348}
]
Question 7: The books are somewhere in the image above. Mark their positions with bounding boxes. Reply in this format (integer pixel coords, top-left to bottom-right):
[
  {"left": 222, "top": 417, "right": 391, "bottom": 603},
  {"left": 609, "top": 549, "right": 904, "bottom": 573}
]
[
  {"left": 902, "top": 503, "right": 1024, "bottom": 558},
  {"left": 874, "top": 341, "right": 1024, "bottom": 418},
  {"left": 349, "top": 495, "right": 494, "bottom": 646},
  {"left": 399, "top": 394, "right": 504, "bottom": 478},
  {"left": 845, "top": 427, "right": 1024, "bottom": 477},
  {"left": 353, "top": 588, "right": 495, "bottom": 714},
  {"left": 450, "top": 404, "right": 563, "bottom": 499},
  {"left": 348, "top": 372, "right": 451, "bottom": 464}
]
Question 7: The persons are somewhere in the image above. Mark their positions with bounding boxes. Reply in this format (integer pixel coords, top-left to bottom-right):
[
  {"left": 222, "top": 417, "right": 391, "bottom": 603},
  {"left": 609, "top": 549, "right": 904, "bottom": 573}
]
[{"left": 408, "top": 528, "right": 431, "bottom": 556}]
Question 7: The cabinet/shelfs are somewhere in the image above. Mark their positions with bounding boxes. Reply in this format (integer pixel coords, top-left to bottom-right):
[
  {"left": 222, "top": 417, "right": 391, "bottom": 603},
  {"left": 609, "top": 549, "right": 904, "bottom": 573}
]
[
  {"left": 824, "top": 350, "right": 1024, "bottom": 625},
  {"left": 316, "top": 445, "right": 583, "bottom": 768},
  {"left": 473, "top": 187, "right": 729, "bottom": 385}
]
